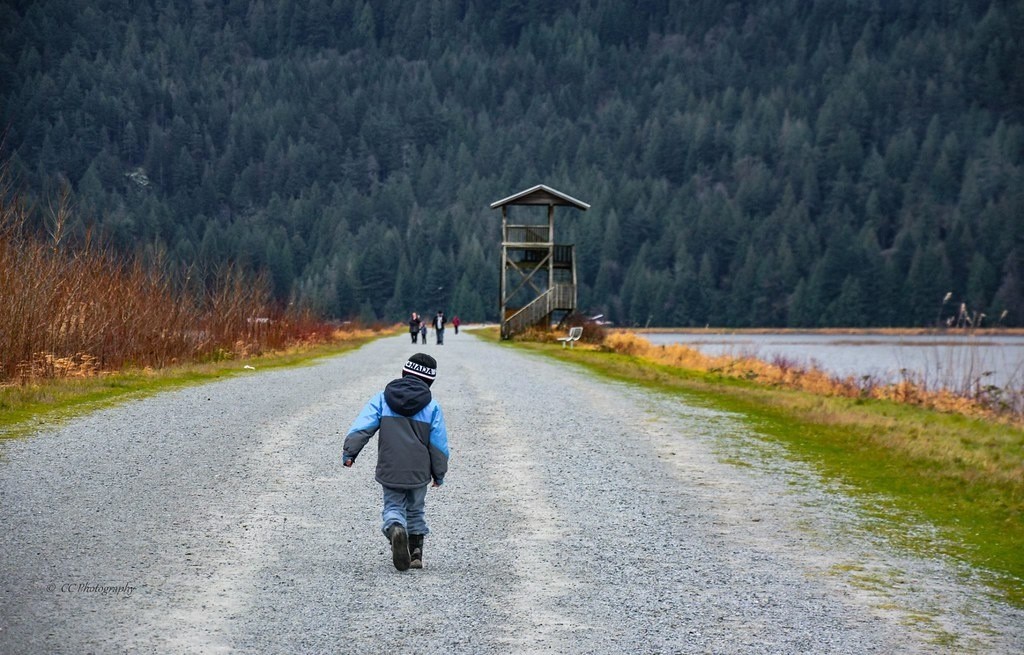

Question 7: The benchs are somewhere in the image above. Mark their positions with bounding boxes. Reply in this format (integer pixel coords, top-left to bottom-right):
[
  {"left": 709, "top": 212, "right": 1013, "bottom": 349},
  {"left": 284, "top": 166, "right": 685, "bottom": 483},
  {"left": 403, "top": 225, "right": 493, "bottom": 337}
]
[{"left": 556, "top": 327, "right": 583, "bottom": 350}]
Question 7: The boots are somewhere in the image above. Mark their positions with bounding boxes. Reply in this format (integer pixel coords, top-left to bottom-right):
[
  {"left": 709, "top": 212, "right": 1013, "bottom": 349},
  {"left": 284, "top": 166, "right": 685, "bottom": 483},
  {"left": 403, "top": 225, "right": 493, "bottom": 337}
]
[
  {"left": 388, "top": 524, "right": 423, "bottom": 571},
  {"left": 422, "top": 338, "right": 426, "bottom": 344}
]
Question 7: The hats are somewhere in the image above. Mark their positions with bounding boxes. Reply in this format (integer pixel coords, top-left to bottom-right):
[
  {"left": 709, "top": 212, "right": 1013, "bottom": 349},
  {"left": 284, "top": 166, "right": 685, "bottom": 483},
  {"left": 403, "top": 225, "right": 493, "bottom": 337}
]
[
  {"left": 402, "top": 352, "right": 437, "bottom": 388},
  {"left": 422, "top": 322, "right": 426, "bottom": 326}
]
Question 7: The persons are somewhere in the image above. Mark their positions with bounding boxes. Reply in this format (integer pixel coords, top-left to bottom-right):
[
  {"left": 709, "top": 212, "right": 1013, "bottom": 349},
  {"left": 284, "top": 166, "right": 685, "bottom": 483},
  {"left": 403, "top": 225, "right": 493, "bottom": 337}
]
[
  {"left": 418, "top": 321, "right": 427, "bottom": 344},
  {"left": 432, "top": 310, "right": 447, "bottom": 345},
  {"left": 452, "top": 316, "right": 460, "bottom": 334},
  {"left": 409, "top": 312, "right": 421, "bottom": 343},
  {"left": 343, "top": 353, "right": 449, "bottom": 571}
]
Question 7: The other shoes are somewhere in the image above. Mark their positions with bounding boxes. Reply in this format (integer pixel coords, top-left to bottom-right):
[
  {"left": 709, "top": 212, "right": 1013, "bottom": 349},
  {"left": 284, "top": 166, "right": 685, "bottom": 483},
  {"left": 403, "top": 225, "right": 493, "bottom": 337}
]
[
  {"left": 437, "top": 343, "right": 443, "bottom": 345},
  {"left": 412, "top": 341, "right": 417, "bottom": 343}
]
